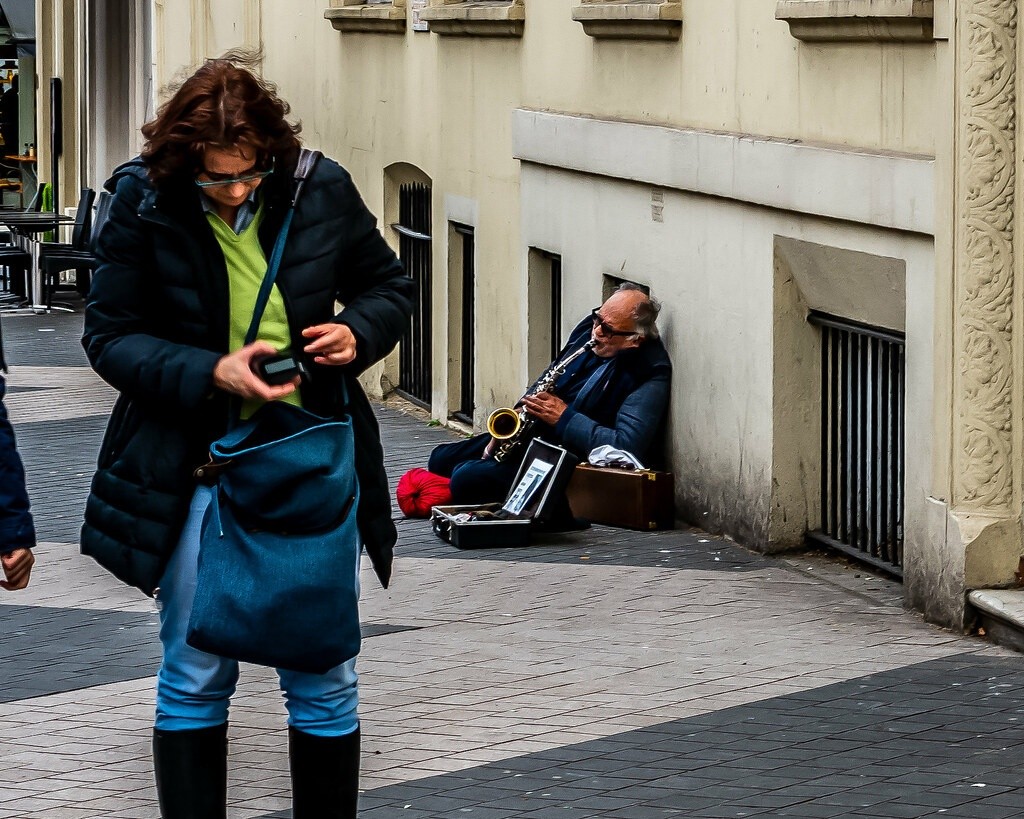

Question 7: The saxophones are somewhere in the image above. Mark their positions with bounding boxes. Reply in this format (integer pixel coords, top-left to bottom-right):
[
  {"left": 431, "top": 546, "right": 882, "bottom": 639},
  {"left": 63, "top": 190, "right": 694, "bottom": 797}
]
[{"left": 487, "top": 336, "right": 602, "bottom": 464}]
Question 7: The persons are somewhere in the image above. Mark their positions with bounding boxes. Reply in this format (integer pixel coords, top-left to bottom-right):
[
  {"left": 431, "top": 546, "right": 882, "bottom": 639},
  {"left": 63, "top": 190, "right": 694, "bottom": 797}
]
[
  {"left": 0, "top": 332, "right": 39, "bottom": 596},
  {"left": 425, "top": 284, "right": 674, "bottom": 507},
  {"left": 74, "top": 59, "right": 418, "bottom": 819}
]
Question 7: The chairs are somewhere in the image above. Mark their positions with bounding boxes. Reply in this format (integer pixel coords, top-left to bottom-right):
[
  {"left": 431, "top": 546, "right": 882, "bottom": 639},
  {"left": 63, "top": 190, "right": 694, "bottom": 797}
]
[
  {"left": 0, "top": 253, "right": 32, "bottom": 308},
  {"left": 0, "top": 163, "right": 24, "bottom": 211},
  {"left": 39, "top": 188, "right": 113, "bottom": 311},
  {"left": 0, "top": 250, "right": 26, "bottom": 304}
]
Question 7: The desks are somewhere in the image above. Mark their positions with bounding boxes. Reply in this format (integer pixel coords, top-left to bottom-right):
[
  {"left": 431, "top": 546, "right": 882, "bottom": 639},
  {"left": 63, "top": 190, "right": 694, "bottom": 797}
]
[
  {"left": 0, "top": 211, "right": 75, "bottom": 314},
  {"left": 5, "top": 155, "right": 37, "bottom": 192}
]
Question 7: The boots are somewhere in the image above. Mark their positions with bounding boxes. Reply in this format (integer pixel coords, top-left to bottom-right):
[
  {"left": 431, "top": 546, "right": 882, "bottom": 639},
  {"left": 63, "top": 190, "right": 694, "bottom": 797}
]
[
  {"left": 149, "top": 722, "right": 233, "bottom": 815},
  {"left": 285, "top": 723, "right": 363, "bottom": 818}
]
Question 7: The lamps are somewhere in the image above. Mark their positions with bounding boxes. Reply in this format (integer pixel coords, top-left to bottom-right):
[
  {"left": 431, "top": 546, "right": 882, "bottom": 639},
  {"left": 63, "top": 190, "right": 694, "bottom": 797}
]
[{"left": 0, "top": 61, "right": 18, "bottom": 69}]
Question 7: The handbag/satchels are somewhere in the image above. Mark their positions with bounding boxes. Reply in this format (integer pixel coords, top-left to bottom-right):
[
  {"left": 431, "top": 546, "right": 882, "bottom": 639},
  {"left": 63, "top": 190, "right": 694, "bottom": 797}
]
[
  {"left": 182, "top": 399, "right": 364, "bottom": 678},
  {"left": 396, "top": 466, "right": 452, "bottom": 520}
]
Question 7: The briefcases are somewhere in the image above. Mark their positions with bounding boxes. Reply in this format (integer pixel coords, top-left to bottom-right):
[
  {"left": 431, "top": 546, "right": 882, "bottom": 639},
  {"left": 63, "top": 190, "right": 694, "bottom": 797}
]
[
  {"left": 429, "top": 438, "right": 575, "bottom": 550},
  {"left": 574, "top": 458, "right": 668, "bottom": 534}
]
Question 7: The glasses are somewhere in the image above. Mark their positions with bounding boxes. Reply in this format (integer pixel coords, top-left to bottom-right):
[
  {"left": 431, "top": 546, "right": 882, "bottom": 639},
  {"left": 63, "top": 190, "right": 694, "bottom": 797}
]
[
  {"left": 591, "top": 306, "right": 639, "bottom": 340},
  {"left": 183, "top": 157, "right": 285, "bottom": 190}
]
